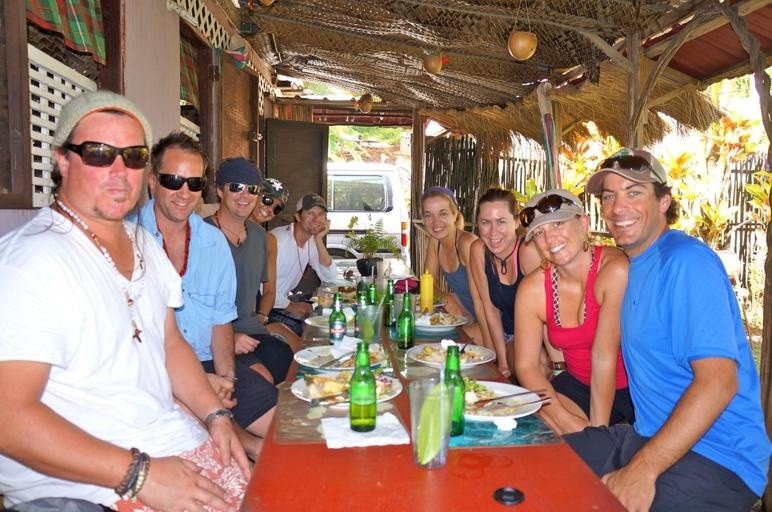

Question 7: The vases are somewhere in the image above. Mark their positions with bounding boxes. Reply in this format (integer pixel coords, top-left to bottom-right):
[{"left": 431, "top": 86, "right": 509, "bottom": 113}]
[
  {"left": 358, "top": 103, "right": 372, "bottom": 114},
  {"left": 423, "top": 55, "right": 442, "bottom": 74},
  {"left": 502, "top": 0, "right": 540, "bottom": 61}
]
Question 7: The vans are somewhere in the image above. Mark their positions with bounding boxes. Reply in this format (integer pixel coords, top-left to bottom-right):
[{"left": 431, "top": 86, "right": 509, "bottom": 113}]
[{"left": 326, "top": 162, "right": 409, "bottom": 258}]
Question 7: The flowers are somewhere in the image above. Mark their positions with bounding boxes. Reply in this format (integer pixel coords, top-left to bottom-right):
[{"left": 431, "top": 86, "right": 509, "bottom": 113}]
[
  {"left": 421, "top": 43, "right": 450, "bottom": 62},
  {"left": 356, "top": 89, "right": 373, "bottom": 104}
]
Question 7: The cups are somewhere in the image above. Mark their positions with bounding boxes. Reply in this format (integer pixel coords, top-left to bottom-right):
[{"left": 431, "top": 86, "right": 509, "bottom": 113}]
[{"left": 408, "top": 374, "right": 454, "bottom": 470}]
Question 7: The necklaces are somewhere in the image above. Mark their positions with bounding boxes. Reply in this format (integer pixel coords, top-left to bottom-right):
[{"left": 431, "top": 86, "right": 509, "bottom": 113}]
[
  {"left": 153, "top": 218, "right": 192, "bottom": 276},
  {"left": 493, "top": 251, "right": 515, "bottom": 275},
  {"left": 56, "top": 199, "right": 147, "bottom": 348},
  {"left": 296, "top": 236, "right": 311, "bottom": 278},
  {"left": 219, "top": 214, "right": 247, "bottom": 245}
]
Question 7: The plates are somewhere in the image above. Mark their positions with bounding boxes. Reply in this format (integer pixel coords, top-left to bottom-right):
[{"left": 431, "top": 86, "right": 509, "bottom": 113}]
[
  {"left": 290, "top": 371, "right": 403, "bottom": 406},
  {"left": 409, "top": 340, "right": 497, "bottom": 371},
  {"left": 302, "top": 315, "right": 356, "bottom": 332},
  {"left": 292, "top": 344, "right": 390, "bottom": 370},
  {"left": 412, "top": 294, "right": 448, "bottom": 310},
  {"left": 460, "top": 380, "right": 544, "bottom": 422},
  {"left": 416, "top": 313, "right": 469, "bottom": 334}
]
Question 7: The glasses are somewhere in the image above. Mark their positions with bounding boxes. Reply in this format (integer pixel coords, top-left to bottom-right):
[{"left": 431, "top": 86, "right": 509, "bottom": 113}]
[
  {"left": 262, "top": 196, "right": 282, "bottom": 215},
  {"left": 519, "top": 195, "right": 578, "bottom": 227},
  {"left": 65, "top": 141, "right": 149, "bottom": 170},
  {"left": 227, "top": 183, "right": 260, "bottom": 194},
  {"left": 343, "top": 269, "right": 353, "bottom": 279},
  {"left": 155, "top": 172, "right": 207, "bottom": 193},
  {"left": 595, "top": 156, "right": 663, "bottom": 183}
]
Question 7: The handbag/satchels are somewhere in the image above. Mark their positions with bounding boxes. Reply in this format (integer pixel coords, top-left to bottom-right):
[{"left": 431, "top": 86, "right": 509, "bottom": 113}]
[
  {"left": 393, "top": 277, "right": 420, "bottom": 293},
  {"left": 287, "top": 263, "right": 321, "bottom": 302}
]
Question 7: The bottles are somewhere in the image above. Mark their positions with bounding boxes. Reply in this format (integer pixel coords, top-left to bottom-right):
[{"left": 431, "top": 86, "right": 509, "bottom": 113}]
[
  {"left": 419, "top": 269, "right": 434, "bottom": 315},
  {"left": 355, "top": 279, "right": 416, "bottom": 350},
  {"left": 328, "top": 293, "right": 347, "bottom": 343},
  {"left": 440, "top": 343, "right": 468, "bottom": 438},
  {"left": 349, "top": 342, "right": 380, "bottom": 434}
]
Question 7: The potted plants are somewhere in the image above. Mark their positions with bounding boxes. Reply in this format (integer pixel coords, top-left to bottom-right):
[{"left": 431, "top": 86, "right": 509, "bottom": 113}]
[{"left": 339, "top": 212, "right": 402, "bottom": 276}]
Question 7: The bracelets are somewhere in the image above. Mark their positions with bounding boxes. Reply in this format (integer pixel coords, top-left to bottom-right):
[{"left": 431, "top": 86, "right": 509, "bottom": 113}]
[
  {"left": 113, "top": 448, "right": 149, "bottom": 502},
  {"left": 548, "top": 360, "right": 566, "bottom": 368},
  {"left": 257, "top": 311, "right": 270, "bottom": 323},
  {"left": 205, "top": 409, "right": 235, "bottom": 427}
]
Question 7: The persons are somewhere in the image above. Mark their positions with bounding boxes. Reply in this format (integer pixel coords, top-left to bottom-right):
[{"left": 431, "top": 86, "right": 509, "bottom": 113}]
[
  {"left": 561, "top": 146, "right": 770, "bottom": 512},
  {"left": 0, "top": 90, "right": 261, "bottom": 512},
  {"left": 127, "top": 129, "right": 281, "bottom": 468},
  {"left": 508, "top": 188, "right": 630, "bottom": 446},
  {"left": 267, "top": 187, "right": 332, "bottom": 329},
  {"left": 469, "top": 185, "right": 565, "bottom": 387},
  {"left": 201, "top": 156, "right": 293, "bottom": 386},
  {"left": 416, "top": 183, "right": 495, "bottom": 366},
  {"left": 249, "top": 174, "right": 304, "bottom": 383}
]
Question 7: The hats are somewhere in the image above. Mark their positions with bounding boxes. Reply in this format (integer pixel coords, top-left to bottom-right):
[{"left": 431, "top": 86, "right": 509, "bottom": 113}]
[
  {"left": 296, "top": 193, "right": 328, "bottom": 212},
  {"left": 524, "top": 188, "right": 584, "bottom": 242},
  {"left": 586, "top": 147, "right": 671, "bottom": 193},
  {"left": 262, "top": 178, "right": 289, "bottom": 206},
  {"left": 51, "top": 90, "right": 153, "bottom": 160},
  {"left": 215, "top": 157, "right": 261, "bottom": 185}
]
